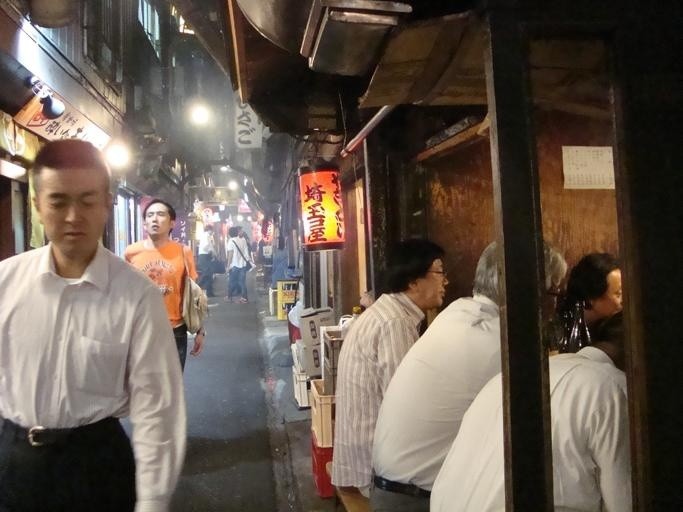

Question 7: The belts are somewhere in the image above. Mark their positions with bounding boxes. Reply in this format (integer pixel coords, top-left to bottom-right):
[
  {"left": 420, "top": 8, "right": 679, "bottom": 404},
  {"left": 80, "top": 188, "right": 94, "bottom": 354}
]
[
  {"left": 373, "top": 475, "right": 431, "bottom": 498},
  {"left": 2, "top": 418, "right": 120, "bottom": 449}
]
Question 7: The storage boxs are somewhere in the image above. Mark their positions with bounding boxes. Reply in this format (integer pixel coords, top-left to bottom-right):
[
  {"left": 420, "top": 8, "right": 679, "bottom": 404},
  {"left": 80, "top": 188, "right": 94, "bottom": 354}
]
[
  {"left": 296, "top": 339, "right": 322, "bottom": 378},
  {"left": 277, "top": 281, "right": 299, "bottom": 321},
  {"left": 324, "top": 329, "right": 344, "bottom": 415},
  {"left": 309, "top": 379, "right": 335, "bottom": 447},
  {"left": 298, "top": 307, "right": 337, "bottom": 346},
  {"left": 312, "top": 432, "right": 335, "bottom": 498}
]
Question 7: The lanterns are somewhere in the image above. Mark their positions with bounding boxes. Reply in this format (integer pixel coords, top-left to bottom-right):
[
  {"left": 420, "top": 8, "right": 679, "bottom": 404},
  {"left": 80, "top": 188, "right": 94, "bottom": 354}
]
[{"left": 262, "top": 221, "right": 274, "bottom": 243}]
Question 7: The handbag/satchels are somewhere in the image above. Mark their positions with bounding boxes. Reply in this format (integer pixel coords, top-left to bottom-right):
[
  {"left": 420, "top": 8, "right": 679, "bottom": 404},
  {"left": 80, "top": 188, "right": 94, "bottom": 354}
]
[
  {"left": 246, "top": 261, "right": 252, "bottom": 270},
  {"left": 181, "top": 276, "right": 202, "bottom": 336},
  {"left": 210, "top": 257, "right": 226, "bottom": 273}
]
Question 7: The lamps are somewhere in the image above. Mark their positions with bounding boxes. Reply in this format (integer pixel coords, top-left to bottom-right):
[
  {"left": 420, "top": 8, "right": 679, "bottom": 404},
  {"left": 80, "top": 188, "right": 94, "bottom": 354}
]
[{"left": 26, "top": 76, "right": 66, "bottom": 120}]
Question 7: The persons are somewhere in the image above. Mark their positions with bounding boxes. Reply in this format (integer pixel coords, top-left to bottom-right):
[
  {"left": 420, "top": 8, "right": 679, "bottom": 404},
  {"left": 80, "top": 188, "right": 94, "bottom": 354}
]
[
  {"left": 0, "top": 140, "right": 186, "bottom": 512},
  {"left": 331, "top": 238, "right": 450, "bottom": 512},
  {"left": 198, "top": 224, "right": 256, "bottom": 303},
  {"left": 566, "top": 252, "right": 623, "bottom": 324},
  {"left": 428, "top": 309, "right": 633, "bottom": 512},
  {"left": 371, "top": 238, "right": 568, "bottom": 512},
  {"left": 124, "top": 200, "right": 208, "bottom": 374}
]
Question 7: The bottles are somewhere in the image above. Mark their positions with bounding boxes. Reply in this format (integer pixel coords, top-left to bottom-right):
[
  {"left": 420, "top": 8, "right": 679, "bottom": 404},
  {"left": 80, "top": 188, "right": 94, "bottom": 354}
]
[
  {"left": 558, "top": 308, "right": 575, "bottom": 353},
  {"left": 568, "top": 303, "right": 593, "bottom": 351}
]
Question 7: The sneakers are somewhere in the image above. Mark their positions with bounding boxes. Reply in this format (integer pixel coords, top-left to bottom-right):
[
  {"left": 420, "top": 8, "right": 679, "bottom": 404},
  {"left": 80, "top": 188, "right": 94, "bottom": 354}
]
[{"left": 235, "top": 298, "right": 247, "bottom": 304}]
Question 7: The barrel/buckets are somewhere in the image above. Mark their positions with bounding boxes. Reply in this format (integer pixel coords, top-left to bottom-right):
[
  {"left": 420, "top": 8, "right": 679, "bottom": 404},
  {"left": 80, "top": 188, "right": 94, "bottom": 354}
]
[{"left": 341, "top": 305, "right": 362, "bottom": 338}]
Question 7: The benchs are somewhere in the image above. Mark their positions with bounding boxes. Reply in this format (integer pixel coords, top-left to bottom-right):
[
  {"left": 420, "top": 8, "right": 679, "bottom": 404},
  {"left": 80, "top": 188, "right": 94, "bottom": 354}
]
[{"left": 326, "top": 461, "right": 375, "bottom": 512}]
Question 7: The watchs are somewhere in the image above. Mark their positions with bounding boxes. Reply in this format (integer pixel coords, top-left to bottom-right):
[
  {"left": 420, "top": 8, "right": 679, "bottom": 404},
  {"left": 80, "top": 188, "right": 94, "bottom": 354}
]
[{"left": 197, "top": 328, "right": 207, "bottom": 336}]
